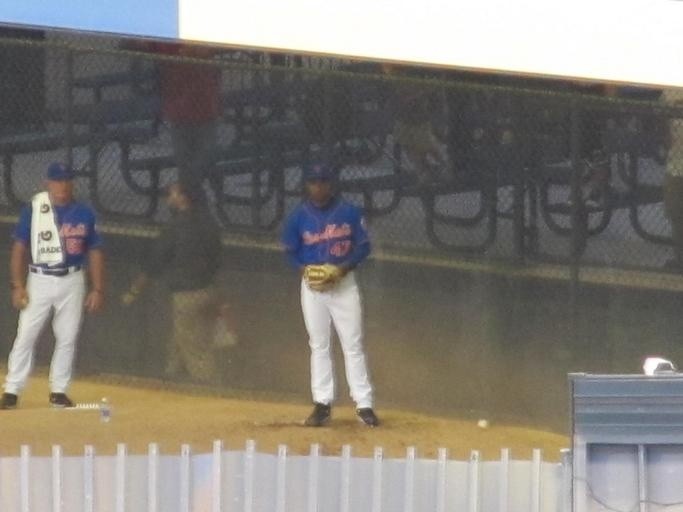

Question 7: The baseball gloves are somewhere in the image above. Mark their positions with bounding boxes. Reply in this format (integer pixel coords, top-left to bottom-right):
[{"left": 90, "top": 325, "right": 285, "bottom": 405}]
[{"left": 303, "top": 263, "right": 344, "bottom": 294}]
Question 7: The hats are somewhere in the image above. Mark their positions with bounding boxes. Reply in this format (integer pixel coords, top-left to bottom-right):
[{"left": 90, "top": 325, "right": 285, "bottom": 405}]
[
  {"left": 46, "top": 162, "right": 77, "bottom": 181},
  {"left": 302, "top": 158, "right": 337, "bottom": 180}
]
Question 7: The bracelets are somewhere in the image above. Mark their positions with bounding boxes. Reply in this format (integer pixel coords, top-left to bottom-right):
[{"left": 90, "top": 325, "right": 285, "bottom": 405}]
[
  {"left": 92, "top": 287, "right": 104, "bottom": 294},
  {"left": 7, "top": 279, "right": 25, "bottom": 290}
]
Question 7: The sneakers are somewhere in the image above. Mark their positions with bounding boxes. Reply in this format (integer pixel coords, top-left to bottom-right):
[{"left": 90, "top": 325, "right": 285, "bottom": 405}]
[
  {"left": 305, "top": 403, "right": 331, "bottom": 426},
  {"left": 355, "top": 408, "right": 378, "bottom": 427},
  {"left": 49, "top": 393, "right": 72, "bottom": 408},
  {"left": 0, "top": 392, "right": 17, "bottom": 410}
]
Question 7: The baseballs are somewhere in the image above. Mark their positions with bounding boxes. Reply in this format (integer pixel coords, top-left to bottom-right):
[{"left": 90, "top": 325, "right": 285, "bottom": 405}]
[
  {"left": 477, "top": 419, "right": 488, "bottom": 430},
  {"left": 21, "top": 298, "right": 27, "bottom": 307},
  {"left": 102, "top": 397, "right": 107, "bottom": 403}
]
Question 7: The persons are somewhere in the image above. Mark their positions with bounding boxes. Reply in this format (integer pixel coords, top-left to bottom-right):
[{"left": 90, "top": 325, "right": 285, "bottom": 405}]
[
  {"left": 648, "top": 88, "right": 682, "bottom": 277},
  {"left": 373, "top": 63, "right": 451, "bottom": 179},
  {"left": 0, "top": 160, "right": 106, "bottom": 407},
  {"left": 279, "top": 162, "right": 382, "bottom": 430},
  {"left": 142, "top": 42, "right": 230, "bottom": 241},
  {"left": 571, "top": 81, "right": 627, "bottom": 130},
  {"left": 117, "top": 177, "right": 233, "bottom": 385}
]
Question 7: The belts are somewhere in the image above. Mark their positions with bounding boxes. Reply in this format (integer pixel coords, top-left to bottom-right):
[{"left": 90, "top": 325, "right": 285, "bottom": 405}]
[{"left": 30, "top": 265, "right": 81, "bottom": 276}]
